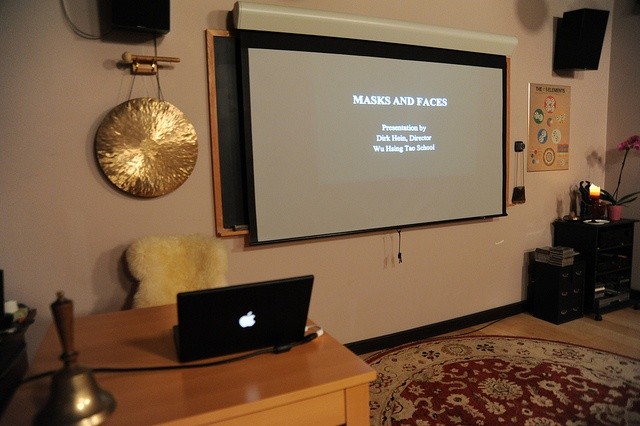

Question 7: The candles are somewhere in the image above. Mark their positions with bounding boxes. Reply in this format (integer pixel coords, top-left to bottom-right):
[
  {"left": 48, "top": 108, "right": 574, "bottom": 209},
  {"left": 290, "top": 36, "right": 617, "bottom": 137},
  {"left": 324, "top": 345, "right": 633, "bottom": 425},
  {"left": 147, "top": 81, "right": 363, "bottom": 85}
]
[{"left": 590, "top": 184, "right": 602, "bottom": 196}]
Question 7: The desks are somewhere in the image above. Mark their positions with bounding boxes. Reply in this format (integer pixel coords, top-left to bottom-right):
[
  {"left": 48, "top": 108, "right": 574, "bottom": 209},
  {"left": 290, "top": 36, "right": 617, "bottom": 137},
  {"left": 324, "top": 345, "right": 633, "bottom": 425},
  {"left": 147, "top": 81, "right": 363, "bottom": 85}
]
[{"left": 2, "top": 301, "right": 379, "bottom": 425}]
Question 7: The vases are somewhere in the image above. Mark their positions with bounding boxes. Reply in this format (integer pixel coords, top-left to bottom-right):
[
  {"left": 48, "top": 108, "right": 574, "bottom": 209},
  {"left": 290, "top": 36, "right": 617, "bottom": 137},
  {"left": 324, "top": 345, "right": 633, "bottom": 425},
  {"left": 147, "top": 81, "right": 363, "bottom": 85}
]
[{"left": 607, "top": 204, "right": 624, "bottom": 220}]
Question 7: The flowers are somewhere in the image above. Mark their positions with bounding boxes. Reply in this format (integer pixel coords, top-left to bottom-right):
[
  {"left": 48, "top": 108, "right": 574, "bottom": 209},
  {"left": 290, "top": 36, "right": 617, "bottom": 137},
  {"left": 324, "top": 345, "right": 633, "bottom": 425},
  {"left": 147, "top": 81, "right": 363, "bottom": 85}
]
[{"left": 600, "top": 134, "right": 640, "bottom": 207}]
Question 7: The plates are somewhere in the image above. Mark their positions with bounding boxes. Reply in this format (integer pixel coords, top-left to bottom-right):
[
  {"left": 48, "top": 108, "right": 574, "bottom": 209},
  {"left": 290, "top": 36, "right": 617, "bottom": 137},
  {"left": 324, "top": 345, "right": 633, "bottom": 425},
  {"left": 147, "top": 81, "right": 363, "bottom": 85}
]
[{"left": 582, "top": 219, "right": 610, "bottom": 225}]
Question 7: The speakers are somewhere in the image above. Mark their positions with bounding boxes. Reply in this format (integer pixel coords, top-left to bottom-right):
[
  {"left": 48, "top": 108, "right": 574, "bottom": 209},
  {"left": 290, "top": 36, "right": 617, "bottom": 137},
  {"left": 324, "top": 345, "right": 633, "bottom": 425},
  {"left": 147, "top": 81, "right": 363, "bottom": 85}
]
[
  {"left": 553, "top": 8, "right": 609, "bottom": 70},
  {"left": 97, "top": 0, "right": 170, "bottom": 44}
]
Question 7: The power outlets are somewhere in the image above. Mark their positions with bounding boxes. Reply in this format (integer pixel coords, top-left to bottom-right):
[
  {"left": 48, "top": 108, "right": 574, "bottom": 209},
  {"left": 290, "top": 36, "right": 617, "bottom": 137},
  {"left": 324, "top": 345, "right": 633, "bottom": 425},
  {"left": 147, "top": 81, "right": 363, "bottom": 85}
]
[{"left": 394, "top": 251, "right": 405, "bottom": 264}]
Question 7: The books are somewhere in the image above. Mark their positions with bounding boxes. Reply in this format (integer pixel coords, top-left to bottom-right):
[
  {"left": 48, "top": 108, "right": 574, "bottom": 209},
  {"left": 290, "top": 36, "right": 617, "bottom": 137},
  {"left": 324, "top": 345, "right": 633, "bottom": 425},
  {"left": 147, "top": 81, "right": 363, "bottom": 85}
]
[{"left": 534, "top": 245, "right": 580, "bottom": 267}]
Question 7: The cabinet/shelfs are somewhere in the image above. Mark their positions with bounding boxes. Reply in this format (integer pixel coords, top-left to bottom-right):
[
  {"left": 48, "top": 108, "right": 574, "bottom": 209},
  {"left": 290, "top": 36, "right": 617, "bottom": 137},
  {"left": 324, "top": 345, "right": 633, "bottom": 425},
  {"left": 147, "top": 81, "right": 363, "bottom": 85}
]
[
  {"left": 527, "top": 247, "right": 587, "bottom": 325},
  {"left": 550, "top": 219, "right": 640, "bottom": 320}
]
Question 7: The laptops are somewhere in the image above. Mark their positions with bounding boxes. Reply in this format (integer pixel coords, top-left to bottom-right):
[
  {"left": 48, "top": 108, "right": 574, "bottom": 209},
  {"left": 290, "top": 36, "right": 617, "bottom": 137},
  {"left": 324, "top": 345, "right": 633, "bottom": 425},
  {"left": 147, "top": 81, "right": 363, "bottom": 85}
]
[{"left": 173, "top": 275, "right": 314, "bottom": 363}]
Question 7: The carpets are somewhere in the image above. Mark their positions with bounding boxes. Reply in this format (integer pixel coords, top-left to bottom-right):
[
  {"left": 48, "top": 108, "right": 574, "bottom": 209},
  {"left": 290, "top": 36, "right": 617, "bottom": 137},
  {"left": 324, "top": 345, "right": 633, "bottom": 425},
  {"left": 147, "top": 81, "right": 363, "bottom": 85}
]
[{"left": 362, "top": 334, "right": 640, "bottom": 425}]
